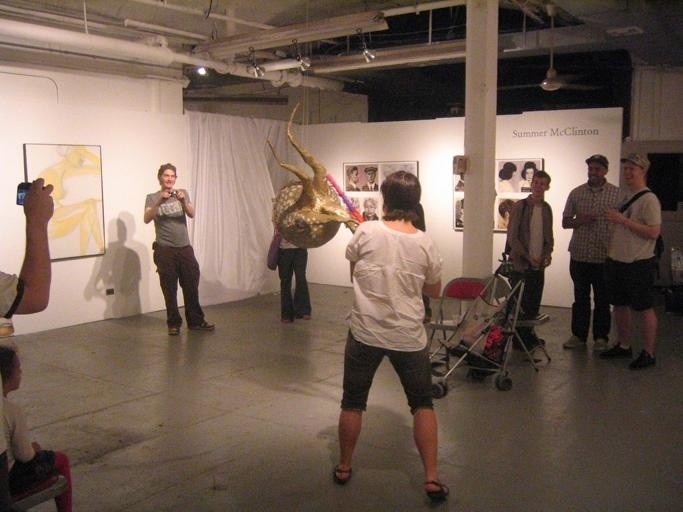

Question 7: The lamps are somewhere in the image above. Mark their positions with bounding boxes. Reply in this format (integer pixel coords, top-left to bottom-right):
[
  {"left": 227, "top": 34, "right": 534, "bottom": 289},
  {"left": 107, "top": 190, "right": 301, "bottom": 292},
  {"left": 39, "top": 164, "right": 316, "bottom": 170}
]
[{"left": 203, "top": 8, "right": 390, "bottom": 83}]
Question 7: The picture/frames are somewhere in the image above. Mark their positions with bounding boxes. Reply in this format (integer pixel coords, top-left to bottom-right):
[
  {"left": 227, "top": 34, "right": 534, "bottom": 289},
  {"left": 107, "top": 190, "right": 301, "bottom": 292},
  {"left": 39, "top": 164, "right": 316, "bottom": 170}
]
[
  {"left": 452, "top": 157, "right": 544, "bottom": 233},
  {"left": 341, "top": 160, "right": 419, "bottom": 222},
  {"left": 21, "top": 142, "right": 106, "bottom": 264}
]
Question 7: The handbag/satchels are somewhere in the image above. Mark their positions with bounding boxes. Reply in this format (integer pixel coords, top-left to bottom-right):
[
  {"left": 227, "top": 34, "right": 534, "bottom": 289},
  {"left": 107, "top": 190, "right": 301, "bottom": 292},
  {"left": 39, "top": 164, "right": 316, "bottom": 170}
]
[
  {"left": 505, "top": 237, "right": 514, "bottom": 256},
  {"left": 654, "top": 235, "right": 665, "bottom": 258}
]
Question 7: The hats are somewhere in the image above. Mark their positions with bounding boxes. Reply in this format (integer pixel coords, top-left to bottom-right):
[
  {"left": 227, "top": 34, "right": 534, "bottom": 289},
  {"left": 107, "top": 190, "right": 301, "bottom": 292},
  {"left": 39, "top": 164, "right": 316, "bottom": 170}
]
[
  {"left": 585, "top": 154, "right": 609, "bottom": 169},
  {"left": 620, "top": 150, "right": 653, "bottom": 170}
]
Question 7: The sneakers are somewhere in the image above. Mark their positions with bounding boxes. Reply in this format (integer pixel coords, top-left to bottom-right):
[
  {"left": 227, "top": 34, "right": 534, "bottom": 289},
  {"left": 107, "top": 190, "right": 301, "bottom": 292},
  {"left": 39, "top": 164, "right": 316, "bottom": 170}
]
[
  {"left": 513, "top": 332, "right": 547, "bottom": 353},
  {"left": 295, "top": 309, "right": 312, "bottom": 320},
  {"left": 561, "top": 335, "right": 587, "bottom": 349},
  {"left": 188, "top": 319, "right": 216, "bottom": 332},
  {"left": 629, "top": 348, "right": 657, "bottom": 371},
  {"left": 281, "top": 309, "right": 297, "bottom": 324},
  {"left": 167, "top": 321, "right": 182, "bottom": 336},
  {"left": 591, "top": 337, "right": 608, "bottom": 351},
  {"left": 599, "top": 341, "right": 634, "bottom": 359}
]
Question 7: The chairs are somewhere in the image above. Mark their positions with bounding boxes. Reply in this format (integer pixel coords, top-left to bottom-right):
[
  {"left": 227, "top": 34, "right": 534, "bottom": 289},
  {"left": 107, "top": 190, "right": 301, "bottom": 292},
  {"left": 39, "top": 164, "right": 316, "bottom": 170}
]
[
  {"left": 426, "top": 279, "right": 488, "bottom": 362},
  {"left": 0, "top": 447, "right": 69, "bottom": 511},
  {"left": 486, "top": 274, "right": 550, "bottom": 371}
]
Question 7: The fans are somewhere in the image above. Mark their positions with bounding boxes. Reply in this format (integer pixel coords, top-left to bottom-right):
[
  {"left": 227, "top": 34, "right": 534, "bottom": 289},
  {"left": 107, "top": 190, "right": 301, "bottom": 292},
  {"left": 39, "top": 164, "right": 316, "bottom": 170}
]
[{"left": 498, "top": 5, "right": 599, "bottom": 93}]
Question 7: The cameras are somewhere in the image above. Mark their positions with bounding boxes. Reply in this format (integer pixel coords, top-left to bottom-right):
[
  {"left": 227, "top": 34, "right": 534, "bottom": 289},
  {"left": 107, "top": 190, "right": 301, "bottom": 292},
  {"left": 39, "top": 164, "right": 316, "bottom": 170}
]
[
  {"left": 17, "top": 182, "right": 32, "bottom": 205},
  {"left": 168, "top": 190, "right": 178, "bottom": 197}
]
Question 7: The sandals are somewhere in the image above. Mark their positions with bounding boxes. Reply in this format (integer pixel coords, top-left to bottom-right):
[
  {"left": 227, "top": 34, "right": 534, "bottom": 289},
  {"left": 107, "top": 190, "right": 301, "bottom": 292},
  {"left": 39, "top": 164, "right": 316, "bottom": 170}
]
[
  {"left": 331, "top": 463, "right": 356, "bottom": 486},
  {"left": 423, "top": 480, "right": 453, "bottom": 505}
]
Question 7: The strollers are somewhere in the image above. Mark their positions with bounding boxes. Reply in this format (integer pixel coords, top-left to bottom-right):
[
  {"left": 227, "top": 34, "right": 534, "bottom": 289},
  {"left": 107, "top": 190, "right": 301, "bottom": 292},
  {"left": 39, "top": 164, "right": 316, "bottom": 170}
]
[{"left": 419, "top": 254, "right": 534, "bottom": 398}]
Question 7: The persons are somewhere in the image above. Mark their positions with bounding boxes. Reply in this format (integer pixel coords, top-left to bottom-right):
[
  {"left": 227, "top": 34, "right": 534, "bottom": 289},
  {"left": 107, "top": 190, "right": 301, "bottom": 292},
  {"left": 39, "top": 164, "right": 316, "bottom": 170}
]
[
  {"left": 332, "top": 169, "right": 450, "bottom": 502},
  {"left": 344, "top": 167, "right": 361, "bottom": 192},
  {"left": 362, "top": 166, "right": 378, "bottom": 192},
  {"left": 0, "top": 177, "right": 55, "bottom": 320},
  {"left": 361, "top": 196, "right": 379, "bottom": 221},
  {"left": 561, "top": 154, "right": 619, "bottom": 352},
  {"left": 518, "top": 162, "right": 538, "bottom": 192},
  {"left": 277, "top": 231, "right": 312, "bottom": 324},
  {"left": 0, "top": 343, "right": 73, "bottom": 512},
  {"left": 598, "top": 153, "right": 664, "bottom": 370},
  {"left": 497, "top": 198, "right": 517, "bottom": 230},
  {"left": 496, "top": 162, "right": 517, "bottom": 192},
  {"left": 142, "top": 161, "right": 216, "bottom": 337},
  {"left": 411, "top": 202, "right": 432, "bottom": 325},
  {"left": 505, "top": 171, "right": 554, "bottom": 352}
]
